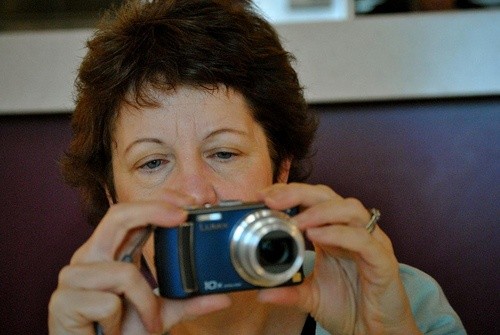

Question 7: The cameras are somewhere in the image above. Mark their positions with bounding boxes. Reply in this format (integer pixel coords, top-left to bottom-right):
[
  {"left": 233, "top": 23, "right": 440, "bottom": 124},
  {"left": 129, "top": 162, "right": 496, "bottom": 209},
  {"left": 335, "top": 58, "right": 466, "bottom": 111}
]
[{"left": 153, "top": 199, "right": 306, "bottom": 300}]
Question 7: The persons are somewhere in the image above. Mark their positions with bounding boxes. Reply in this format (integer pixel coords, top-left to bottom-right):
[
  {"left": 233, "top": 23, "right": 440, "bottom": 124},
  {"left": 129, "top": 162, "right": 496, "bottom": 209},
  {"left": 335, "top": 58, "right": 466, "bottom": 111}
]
[{"left": 48, "top": 0, "right": 466, "bottom": 335}]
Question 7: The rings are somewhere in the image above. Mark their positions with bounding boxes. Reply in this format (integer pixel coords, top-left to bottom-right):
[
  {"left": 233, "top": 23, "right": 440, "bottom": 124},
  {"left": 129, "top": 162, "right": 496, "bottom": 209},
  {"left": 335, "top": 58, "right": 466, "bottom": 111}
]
[{"left": 365, "top": 207, "right": 381, "bottom": 235}]
